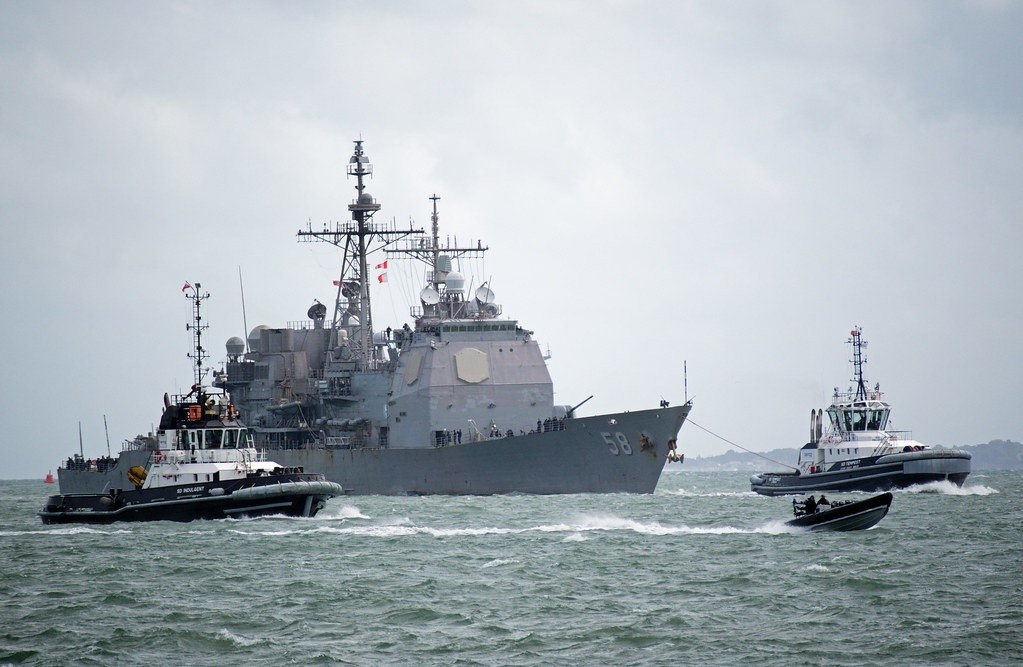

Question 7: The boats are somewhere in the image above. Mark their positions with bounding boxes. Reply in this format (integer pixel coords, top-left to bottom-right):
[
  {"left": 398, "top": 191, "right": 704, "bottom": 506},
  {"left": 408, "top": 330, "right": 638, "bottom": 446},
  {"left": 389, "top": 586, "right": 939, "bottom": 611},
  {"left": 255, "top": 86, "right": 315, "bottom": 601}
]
[
  {"left": 214, "top": 132, "right": 696, "bottom": 495},
  {"left": 748, "top": 326, "right": 973, "bottom": 497},
  {"left": 782, "top": 489, "right": 891, "bottom": 536},
  {"left": 39, "top": 282, "right": 343, "bottom": 521}
]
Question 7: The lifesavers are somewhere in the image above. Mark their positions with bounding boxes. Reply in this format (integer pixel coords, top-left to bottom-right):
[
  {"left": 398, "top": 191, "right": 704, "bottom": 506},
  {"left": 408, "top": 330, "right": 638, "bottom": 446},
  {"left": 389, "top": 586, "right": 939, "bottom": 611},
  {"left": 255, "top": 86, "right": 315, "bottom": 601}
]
[
  {"left": 188, "top": 405, "right": 201, "bottom": 421},
  {"left": 155, "top": 451, "right": 162, "bottom": 462},
  {"left": 231, "top": 404, "right": 235, "bottom": 415}
]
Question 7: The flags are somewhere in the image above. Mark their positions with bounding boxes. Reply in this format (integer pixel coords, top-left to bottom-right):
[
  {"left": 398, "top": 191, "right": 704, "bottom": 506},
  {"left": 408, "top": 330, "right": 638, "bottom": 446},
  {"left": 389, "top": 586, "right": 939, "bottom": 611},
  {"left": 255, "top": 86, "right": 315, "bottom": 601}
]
[
  {"left": 378, "top": 272, "right": 388, "bottom": 283},
  {"left": 375, "top": 261, "right": 387, "bottom": 269}
]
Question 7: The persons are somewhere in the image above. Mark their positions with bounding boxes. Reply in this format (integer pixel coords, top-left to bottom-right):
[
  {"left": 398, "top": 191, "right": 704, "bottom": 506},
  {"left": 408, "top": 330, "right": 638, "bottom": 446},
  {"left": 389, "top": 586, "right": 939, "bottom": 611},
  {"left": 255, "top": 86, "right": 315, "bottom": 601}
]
[
  {"left": 457, "top": 429, "right": 462, "bottom": 444},
  {"left": 490, "top": 424, "right": 501, "bottom": 437},
  {"left": 559, "top": 415, "right": 567, "bottom": 430},
  {"left": 552, "top": 416, "right": 559, "bottom": 431},
  {"left": 801, "top": 496, "right": 816, "bottom": 515},
  {"left": 817, "top": 495, "right": 829, "bottom": 506},
  {"left": 66, "top": 457, "right": 92, "bottom": 471},
  {"left": 537, "top": 419, "right": 541, "bottom": 433},
  {"left": 543, "top": 416, "right": 552, "bottom": 432},
  {"left": 452, "top": 430, "right": 456, "bottom": 445},
  {"left": 96, "top": 456, "right": 109, "bottom": 473},
  {"left": 437, "top": 431, "right": 451, "bottom": 448}
]
